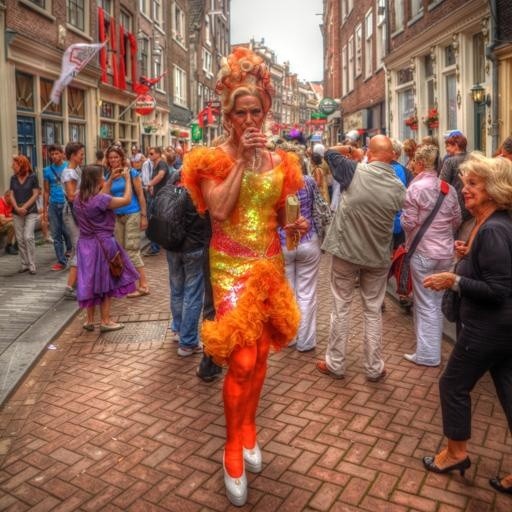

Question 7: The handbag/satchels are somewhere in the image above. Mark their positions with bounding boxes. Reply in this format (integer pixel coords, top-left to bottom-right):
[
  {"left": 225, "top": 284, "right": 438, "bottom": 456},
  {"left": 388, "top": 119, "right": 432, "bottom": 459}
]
[
  {"left": 387, "top": 244, "right": 412, "bottom": 296},
  {"left": 110, "top": 250, "right": 123, "bottom": 278},
  {"left": 312, "top": 190, "right": 333, "bottom": 239},
  {"left": 441, "top": 289, "right": 458, "bottom": 322}
]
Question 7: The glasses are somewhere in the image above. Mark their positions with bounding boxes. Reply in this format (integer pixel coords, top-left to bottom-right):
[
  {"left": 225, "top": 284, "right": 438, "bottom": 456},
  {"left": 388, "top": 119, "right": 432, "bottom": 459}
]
[{"left": 150, "top": 153, "right": 153, "bottom": 156}]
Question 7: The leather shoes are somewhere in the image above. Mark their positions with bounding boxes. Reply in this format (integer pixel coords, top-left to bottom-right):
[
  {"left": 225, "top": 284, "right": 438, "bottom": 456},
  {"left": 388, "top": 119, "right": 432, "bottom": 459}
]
[{"left": 403, "top": 353, "right": 417, "bottom": 363}]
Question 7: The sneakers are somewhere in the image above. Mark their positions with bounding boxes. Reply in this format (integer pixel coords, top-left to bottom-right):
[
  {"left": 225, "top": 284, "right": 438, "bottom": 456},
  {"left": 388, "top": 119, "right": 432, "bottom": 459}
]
[
  {"left": 5, "top": 244, "right": 18, "bottom": 255},
  {"left": 50, "top": 264, "right": 64, "bottom": 271},
  {"left": 30, "top": 271, "right": 36, "bottom": 275},
  {"left": 101, "top": 323, "right": 125, "bottom": 333},
  {"left": 18, "top": 269, "right": 28, "bottom": 273},
  {"left": 316, "top": 360, "right": 345, "bottom": 379},
  {"left": 144, "top": 248, "right": 161, "bottom": 257},
  {"left": 83, "top": 321, "right": 94, "bottom": 331},
  {"left": 173, "top": 331, "right": 180, "bottom": 342},
  {"left": 64, "top": 287, "right": 78, "bottom": 300},
  {"left": 178, "top": 340, "right": 204, "bottom": 356},
  {"left": 368, "top": 368, "right": 386, "bottom": 382},
  {"left": 127, "top": 287, "right": 151, "bottom": 298}
]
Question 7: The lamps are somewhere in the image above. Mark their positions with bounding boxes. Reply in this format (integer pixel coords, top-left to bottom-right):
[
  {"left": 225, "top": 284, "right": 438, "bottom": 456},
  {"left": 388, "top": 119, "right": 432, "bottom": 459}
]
[{"left": 470, "top": 80, "right": 491, "bottom": 108}]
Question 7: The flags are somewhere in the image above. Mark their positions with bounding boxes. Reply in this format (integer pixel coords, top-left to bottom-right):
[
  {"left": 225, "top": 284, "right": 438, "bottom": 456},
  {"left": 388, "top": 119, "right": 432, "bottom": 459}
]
[
  {"left": 50, "top": 41, "right": 101, "bottom": 104},
  {"left": 133, "top": 74, "right": 162, "bottom": 96}
]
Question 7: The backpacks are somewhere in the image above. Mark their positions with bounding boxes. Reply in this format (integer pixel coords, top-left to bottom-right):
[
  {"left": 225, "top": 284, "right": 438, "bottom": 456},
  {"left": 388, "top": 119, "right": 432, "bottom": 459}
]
[{"left": 145, "top": 172, "right": 209, "bottom": 253}]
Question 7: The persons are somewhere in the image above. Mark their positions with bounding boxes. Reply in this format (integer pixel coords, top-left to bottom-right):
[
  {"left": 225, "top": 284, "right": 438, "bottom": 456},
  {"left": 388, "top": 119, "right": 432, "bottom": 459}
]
[
  {"left": 422, "top": 152, "right": 512, "bottom": 500},
  {"left": 275, "top": 129, "right": 512, "bottom": 380},
  {"left": 8, "top": 141, "right": 209, "bottom": 356},
  {"left": 181, "top": 85, "right": 313, "bottom": 507}
]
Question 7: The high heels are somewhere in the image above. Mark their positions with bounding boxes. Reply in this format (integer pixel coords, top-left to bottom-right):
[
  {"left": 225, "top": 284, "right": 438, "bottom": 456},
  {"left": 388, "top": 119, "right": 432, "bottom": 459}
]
[
  {"left": 490, "top": 476, "right": 512, "bottom": 493},
  {"left": 243, "top": 441, "right": 262, "bottom": 473},
  {"left": 423, "top": 456, "right": 471, "bottom": 477},
  {"left": 223, "top": 449, "right": 248, "bottom": 507}
]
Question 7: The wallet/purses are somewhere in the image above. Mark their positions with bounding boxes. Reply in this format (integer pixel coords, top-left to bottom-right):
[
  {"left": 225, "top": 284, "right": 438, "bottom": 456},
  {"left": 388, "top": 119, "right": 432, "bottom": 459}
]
[{"left": 285, "top": 194, "right": 301, "bottom": 251}]
